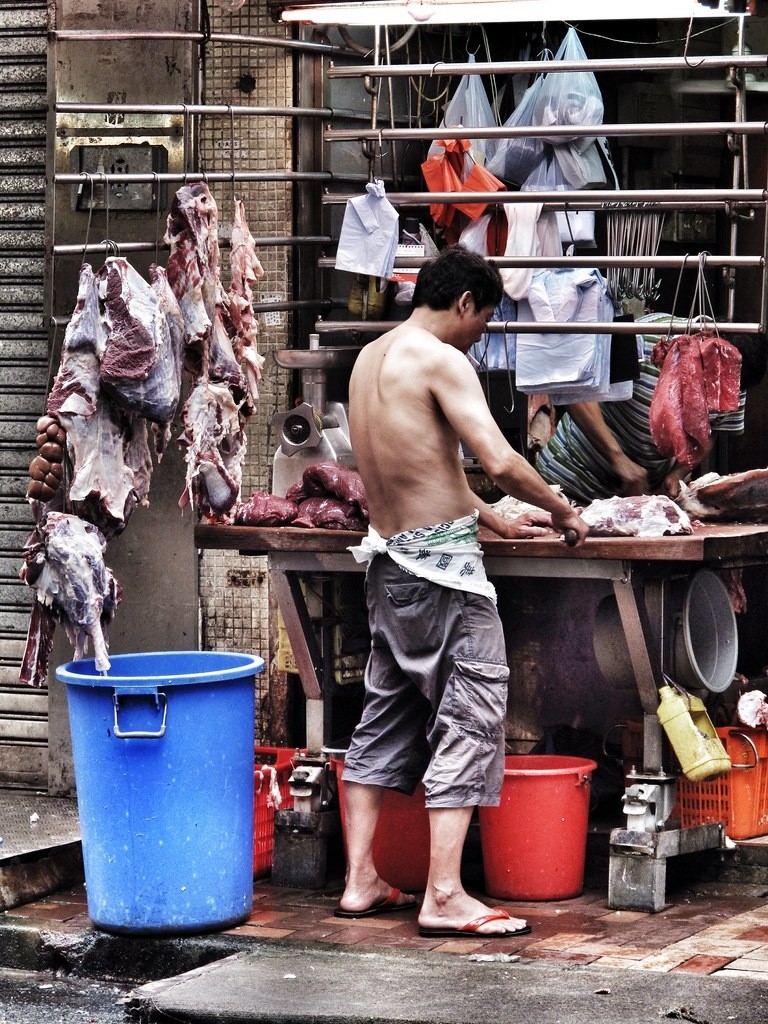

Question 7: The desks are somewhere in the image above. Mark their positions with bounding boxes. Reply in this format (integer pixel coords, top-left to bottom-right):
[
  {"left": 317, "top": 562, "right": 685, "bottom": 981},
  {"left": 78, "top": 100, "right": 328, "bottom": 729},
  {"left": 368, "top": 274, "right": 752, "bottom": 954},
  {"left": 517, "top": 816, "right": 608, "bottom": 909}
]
[{"left": 194, "top": 510, "right": 768, "bottom": 914}]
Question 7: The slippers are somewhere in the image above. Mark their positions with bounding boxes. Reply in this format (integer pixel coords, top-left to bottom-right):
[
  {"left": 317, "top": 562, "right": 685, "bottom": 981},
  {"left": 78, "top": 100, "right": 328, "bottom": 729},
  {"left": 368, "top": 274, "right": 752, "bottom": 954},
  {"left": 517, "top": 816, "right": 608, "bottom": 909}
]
[
  {"left": 333, "top": 888, "right": 418, "bottom": 920},
  {"left": 418, "top": 908, "right": 532, "bottom": 938}
]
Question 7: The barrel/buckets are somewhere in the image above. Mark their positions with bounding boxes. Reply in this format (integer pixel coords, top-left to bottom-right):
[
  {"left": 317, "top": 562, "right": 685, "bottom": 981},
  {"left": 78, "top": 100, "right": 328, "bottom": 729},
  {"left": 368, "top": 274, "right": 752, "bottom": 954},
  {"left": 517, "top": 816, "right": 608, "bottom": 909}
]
[
  {"left": 55, "top": 651, "right": 262, "bottom": 932},
  {"left": 593, "top": 571, "right": 738, "bottom": 693},
  {"left": 480, "top": 755, "right": 597, "bottom": 902},
  {"left": 320, "top": 745, "right": 429, "bottom": 893}
]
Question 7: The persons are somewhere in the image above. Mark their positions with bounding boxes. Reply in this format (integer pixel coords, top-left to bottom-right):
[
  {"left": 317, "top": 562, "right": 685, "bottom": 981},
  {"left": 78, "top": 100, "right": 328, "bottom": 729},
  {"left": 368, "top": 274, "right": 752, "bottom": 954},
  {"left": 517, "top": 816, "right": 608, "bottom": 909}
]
[
  {"left": 529, "top": 309, "right": 746, "bottom": 519},
  {"left": 333, "top": 247, "right": 592, "bottom": 938}
]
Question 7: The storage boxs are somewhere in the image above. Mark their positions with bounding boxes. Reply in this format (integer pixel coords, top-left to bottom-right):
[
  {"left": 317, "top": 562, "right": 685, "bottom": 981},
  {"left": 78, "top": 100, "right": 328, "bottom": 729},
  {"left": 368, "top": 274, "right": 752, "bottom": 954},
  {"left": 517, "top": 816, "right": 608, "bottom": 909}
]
[
  {"left": 602, "top": 720, "right": 768, "bottom": 840},
  {"left": 254, "top": 746, "right": 306, "bottom": 877}
]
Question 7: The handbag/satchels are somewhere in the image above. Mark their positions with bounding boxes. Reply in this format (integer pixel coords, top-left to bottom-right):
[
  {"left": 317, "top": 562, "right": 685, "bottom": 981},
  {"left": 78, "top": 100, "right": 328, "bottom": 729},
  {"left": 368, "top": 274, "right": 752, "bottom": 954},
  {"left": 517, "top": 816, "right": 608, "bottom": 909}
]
[
  {"left": 427, "top": 54, "right": 497, "bottom": 166},
  {"left": 486, "top": 27, "right": 609, "bottom": 252}
]
